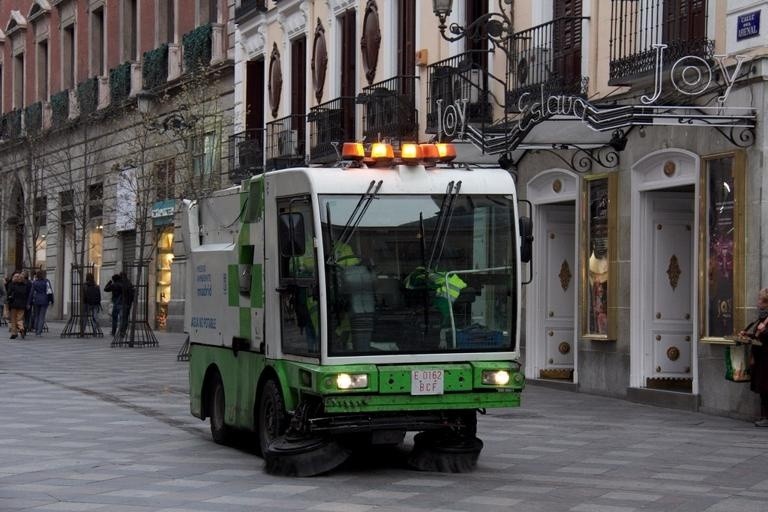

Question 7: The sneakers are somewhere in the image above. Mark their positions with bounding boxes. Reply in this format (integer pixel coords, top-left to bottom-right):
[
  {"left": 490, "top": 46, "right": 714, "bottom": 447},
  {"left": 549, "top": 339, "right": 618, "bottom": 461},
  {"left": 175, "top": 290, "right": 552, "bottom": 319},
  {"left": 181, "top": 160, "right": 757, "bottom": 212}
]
[
  {"left": 754, "top": 418, "right": 768, "bottom": 428},
  {"left": 9, "top": 328, "right": 26, "bottom": 339}
]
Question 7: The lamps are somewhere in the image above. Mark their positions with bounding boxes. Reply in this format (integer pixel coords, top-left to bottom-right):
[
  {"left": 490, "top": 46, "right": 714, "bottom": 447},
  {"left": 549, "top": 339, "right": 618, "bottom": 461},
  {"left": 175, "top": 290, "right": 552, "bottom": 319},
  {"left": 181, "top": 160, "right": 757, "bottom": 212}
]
[
  {"left": 135, "top": 81, "right": 218, "bottom": 136},
  {"left": 432, "top": 0, "right": 513, "bottom": 50}
]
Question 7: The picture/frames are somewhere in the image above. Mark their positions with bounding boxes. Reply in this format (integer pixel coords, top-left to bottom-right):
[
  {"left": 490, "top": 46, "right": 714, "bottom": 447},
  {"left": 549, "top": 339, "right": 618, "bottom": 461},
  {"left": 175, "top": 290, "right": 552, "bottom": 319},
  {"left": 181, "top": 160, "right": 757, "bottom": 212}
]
[
  {"left": 698, "top": 147, "right": 746, "bottom": 343},
  {"left": 581, "top": 173, "right": 619, "bottom": 343}
]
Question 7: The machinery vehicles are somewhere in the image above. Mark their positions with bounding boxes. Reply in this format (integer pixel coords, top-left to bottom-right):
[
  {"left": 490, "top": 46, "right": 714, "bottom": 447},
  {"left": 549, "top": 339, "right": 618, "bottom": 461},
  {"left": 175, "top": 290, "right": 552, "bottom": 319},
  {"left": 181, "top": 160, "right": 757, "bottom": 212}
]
[{"left": 174, "top": 137, "right": 538, "bottom": 480}]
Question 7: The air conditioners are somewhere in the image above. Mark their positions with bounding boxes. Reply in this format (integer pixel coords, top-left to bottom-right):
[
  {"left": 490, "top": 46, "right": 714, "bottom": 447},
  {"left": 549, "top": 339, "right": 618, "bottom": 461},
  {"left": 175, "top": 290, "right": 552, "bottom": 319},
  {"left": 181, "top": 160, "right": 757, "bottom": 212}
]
[
  {"left": 363, "top": 69, "right": 483, "bottom": 133},
  {"left": 275, "top": 108, "right": 343, "bottom": 158},
  {"left": 513, "top": 48, "right": 553, "bottom": 91}
]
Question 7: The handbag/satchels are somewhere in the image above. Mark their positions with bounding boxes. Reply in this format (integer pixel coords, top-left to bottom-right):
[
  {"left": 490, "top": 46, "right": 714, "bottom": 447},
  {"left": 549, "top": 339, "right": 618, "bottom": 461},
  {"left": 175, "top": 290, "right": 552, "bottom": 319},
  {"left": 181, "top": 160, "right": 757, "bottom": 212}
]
[{"left": 723, "top": 340, "right": 755, "bottom": 383}]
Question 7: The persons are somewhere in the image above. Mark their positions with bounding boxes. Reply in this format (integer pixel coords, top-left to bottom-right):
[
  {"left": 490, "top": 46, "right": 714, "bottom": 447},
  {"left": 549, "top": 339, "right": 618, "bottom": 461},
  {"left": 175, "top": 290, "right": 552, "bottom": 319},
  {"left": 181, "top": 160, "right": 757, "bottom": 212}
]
[
  {"left": 118, "top": 271, "right": 136, "bottom": 335},
  {"left": 0, "top": 269, "right": 55, "bottom": 341},
  {"left": 401, "top": 251, "right": 469, "bottom": 352},
  {"left": 281, "top": 204, "right": 363, "bottom": 351},
  {"left": 103, "top": 274, "right": 128, "bottom": 338},
  {"left": 592, "top": 275, "right": 606, "bottom": 335},
  {"left": 76, "top": 272, "right": 104, "bottom": 338},
  {"left": 737, "top": 287, "right": 768, "bottom": 427}
]
